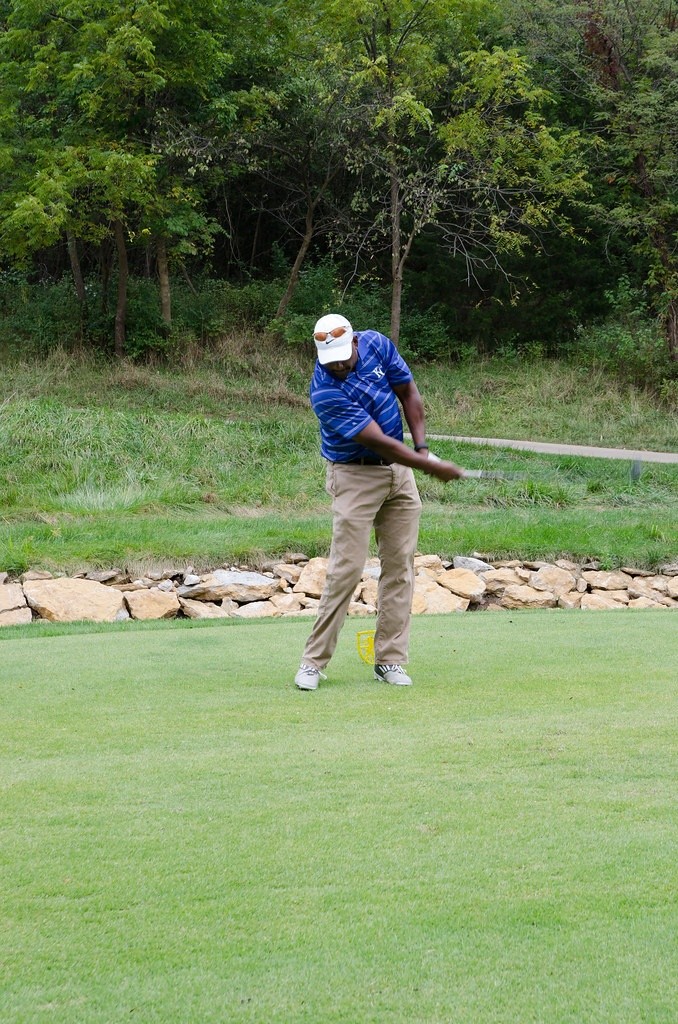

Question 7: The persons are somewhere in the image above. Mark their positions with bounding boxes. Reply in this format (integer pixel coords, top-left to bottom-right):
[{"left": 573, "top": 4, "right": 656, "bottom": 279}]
[{"left": 294, "top": 314, "right": 461, "bottom": 690}]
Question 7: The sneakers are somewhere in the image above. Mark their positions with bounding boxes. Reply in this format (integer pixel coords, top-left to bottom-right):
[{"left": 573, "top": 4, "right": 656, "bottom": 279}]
[
  {"left": 374, "top": 664, "right": 412, "bottom": 686},
  {"left": 295, "top": 663, "right": 328, "bottom": 690}
]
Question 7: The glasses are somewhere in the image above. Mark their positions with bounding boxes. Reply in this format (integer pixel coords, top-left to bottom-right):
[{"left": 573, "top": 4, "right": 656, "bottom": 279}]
[{"left": 312, "top": 326, "right": 348, "bottom": 341}]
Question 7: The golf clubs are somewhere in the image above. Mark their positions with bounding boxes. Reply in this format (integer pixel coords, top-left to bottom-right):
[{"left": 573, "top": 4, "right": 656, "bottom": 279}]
[{"left": 462, "top": 459, "right": 642, "bottom": 487}]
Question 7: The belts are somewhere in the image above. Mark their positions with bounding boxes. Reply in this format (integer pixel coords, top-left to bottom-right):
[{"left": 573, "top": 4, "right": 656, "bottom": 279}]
[{"left": 350, "top": 457, "right": 392, "bottom": 466}]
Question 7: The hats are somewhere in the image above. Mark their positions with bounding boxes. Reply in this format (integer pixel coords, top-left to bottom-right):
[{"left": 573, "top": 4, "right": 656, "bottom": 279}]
[{"left": 314, "top": 314, "right": 353, "bottom": 365}]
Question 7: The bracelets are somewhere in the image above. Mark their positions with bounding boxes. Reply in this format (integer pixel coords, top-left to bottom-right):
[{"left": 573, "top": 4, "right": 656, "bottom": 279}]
[{"left": 414, "top": 444, "right": 428, "bottom": 452}]
